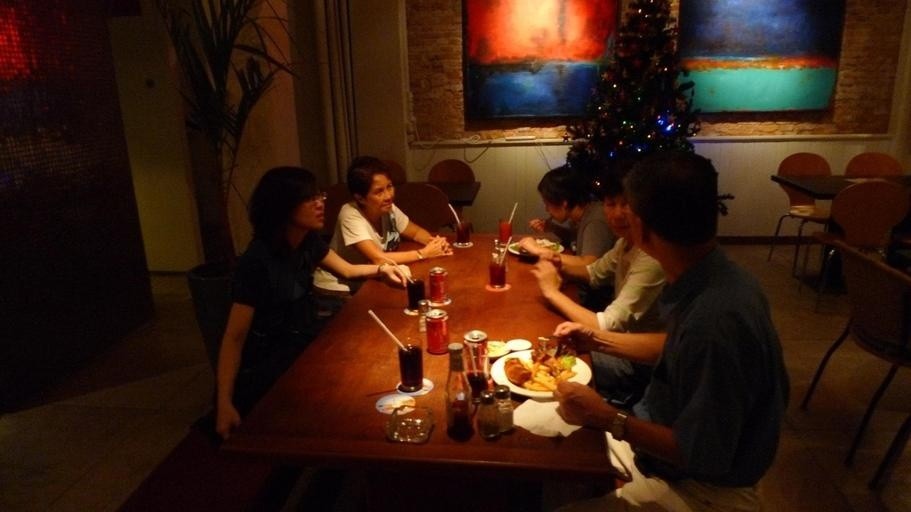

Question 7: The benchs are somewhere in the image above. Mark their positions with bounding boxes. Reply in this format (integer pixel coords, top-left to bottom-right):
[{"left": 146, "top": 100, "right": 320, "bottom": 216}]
[{"left": 118, "top": 404, "right": 304, "bottom": 510}]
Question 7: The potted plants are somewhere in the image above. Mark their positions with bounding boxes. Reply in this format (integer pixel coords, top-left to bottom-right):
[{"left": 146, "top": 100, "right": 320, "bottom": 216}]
[{"left": 157, "top": 0, "right": 305, "bottom": 375}]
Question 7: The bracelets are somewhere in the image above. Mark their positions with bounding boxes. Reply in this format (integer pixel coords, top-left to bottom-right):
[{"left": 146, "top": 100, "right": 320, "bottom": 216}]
[
  {"left": 377, "top": 262, "right": 388, "bottom": 277},
  {"left": 416, "top": 250, "right": 424, "bottom": 261}
]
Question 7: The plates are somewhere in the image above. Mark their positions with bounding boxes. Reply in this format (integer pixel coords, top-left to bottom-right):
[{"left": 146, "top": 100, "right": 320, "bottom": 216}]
[
  {"left": 507, "top": 239, "right": 565, "bottom": 256},
  {"left": 507, "top": 339, "right": 532, "bottom": 352},
  {"left": 491, "top": 351, "right": 593, "bottom": 398},
  {"left": 487, "top": 340, "right": 510, "bottom": 359}
]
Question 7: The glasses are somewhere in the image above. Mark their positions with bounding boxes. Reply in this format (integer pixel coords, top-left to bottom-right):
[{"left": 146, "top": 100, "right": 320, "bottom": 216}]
[{"left": 308, "top": 191, "right": 329, "bottom": 203}]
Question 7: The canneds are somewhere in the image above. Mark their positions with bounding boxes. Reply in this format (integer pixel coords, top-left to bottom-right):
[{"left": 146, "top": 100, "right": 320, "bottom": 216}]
[
  {"left": 429, "top": 267, "right": 449, "bottom": 304},
  {"left": 462, "top": 329, "right": 489, "bottom": 380},
  {"left": 425, "top": 308, "right": 450, "bottom": 355}
]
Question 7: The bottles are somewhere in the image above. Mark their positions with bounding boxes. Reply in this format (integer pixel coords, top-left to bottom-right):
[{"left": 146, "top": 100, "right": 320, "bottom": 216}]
[
  {"left": 417, "top": 299, "right": 430, "bottom": 332},
  {"left": 444, "top": 342, "right": 475, "bottom": 443},
  {"left": 493, "top": 385, "right": 513, "bottom": 434},
  {"left": 478, "top": 389, "right": 501, "bottom": 440}
]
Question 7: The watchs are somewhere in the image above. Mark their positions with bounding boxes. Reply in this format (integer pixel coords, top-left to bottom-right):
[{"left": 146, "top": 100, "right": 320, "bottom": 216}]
[{"left": 611, "top": 410, "right": 629, "bottom": 443}]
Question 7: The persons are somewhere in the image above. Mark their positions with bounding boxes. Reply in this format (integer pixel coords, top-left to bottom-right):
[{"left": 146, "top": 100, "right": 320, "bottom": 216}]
[
  {"left": 546, "top": 151, "right": 791, "bottom": 511},
  {"left": 313, "top": 155, "right": 453, "bottom": 326},
  {"left": 518, "top": 161, "right": 614, "bottom": 311},
  {"left": 214, "top": 165, "right": 414, "bottom": 441},
  {"left": 529, "top": 164, "right": 668, "bottom": 403}
]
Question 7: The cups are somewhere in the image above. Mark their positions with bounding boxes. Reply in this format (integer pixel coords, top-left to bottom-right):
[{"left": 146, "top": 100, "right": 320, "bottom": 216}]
[
  {"left": 456, "top": 218, "right": 472, "bottom": 246},
  {"left": 407, "top": 273, "right": 425, "bottom": 310},
  {"left": 399, "top": 337, "right": 423, "bottom": 392},
  {"left": 498, "top": 217, "right": 514, "bottom": 245},
  {"left": 489, "top": 247, "right": 506, "bottom": 288}
]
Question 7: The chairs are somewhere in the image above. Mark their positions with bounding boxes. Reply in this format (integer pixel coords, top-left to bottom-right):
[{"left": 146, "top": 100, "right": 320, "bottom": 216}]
[
  {"left": 387, "top": 160, "right": 482, "bottom": 230},
  {"left": 319, "top": 186, "right": 352, "bottom": 248}
]
